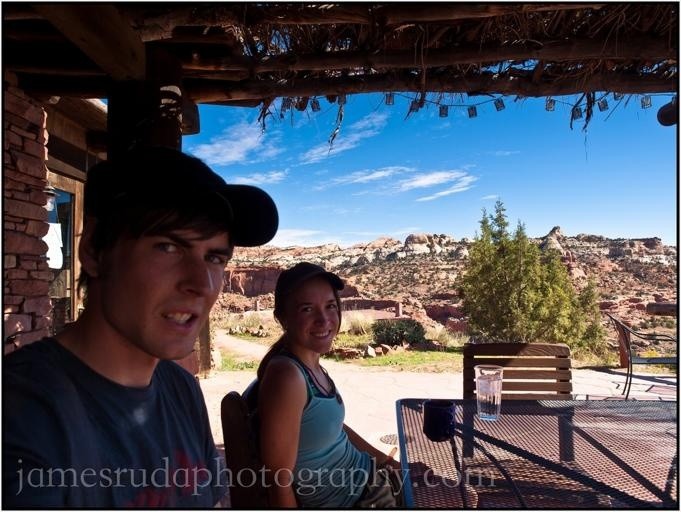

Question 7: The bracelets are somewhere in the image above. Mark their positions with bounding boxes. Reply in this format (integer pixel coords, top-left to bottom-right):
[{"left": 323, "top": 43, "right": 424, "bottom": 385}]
[{"left": 385, "top": 447, "right": 398, "bottom": 465}]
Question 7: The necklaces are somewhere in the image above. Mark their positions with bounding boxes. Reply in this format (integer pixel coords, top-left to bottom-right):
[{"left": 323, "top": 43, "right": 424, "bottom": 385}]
[{"left": 301, "top": 362, "right": 343, "bottom": 404}]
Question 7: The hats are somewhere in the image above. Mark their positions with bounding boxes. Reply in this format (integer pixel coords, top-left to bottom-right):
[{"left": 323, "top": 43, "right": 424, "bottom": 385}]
[
  {"left": 276, "top": 263, "right": 344, "bottom": 290},
  {"left": 84, "top": 146, "right": 278, "bottom": 247}
]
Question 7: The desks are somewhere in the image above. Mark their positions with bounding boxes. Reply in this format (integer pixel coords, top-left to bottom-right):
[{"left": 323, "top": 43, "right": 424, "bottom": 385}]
[{"left": 395, "top": 397, "right": 679, "bottom": 510}]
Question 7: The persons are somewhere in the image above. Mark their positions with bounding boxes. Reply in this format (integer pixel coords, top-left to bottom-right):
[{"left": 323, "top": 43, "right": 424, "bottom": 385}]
[
  {"left": 1, "top": 147, "right": 279, "bottom": 509},
  {"left": 245, "top": 264, "right": 405, "bottom": 509}
]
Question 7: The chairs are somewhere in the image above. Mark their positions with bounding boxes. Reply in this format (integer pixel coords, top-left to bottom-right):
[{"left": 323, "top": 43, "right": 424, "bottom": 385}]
[
  {"left": 221, "top": 392, "right": 265, "bottom": 508},
  {"left": 462, "top": 340, "right": 612, "bottom": 510},
  {"left": 606, "top": 313, "right": 677, "bottom": 402}
]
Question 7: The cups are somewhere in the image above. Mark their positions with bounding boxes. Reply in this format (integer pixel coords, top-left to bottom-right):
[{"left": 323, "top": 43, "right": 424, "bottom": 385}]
[
  {"left": 474, "top": 364, "right": 504, "bottom": 421},
  {"left": 422, "top": 400, "right": 456, "bottom": 442}
]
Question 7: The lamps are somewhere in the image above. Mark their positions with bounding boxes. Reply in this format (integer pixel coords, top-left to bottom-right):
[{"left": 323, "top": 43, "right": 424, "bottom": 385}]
[{"left": 281, "top": 88, "right": 652, "bottom": 119}]
